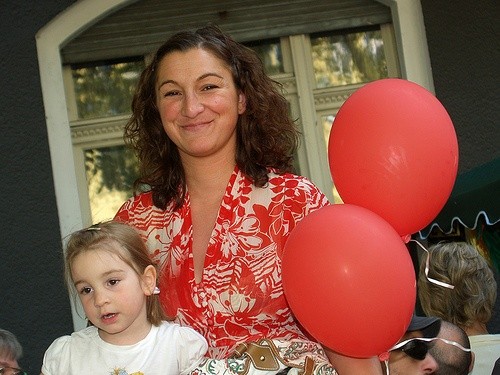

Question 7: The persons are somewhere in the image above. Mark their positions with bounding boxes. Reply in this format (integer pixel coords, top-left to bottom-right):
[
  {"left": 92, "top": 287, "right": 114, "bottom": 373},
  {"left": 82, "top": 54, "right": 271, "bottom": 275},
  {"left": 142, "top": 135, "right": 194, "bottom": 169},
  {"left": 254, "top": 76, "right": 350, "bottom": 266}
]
[
  {"left": 417, "top": 240, "right": 500, "bottom": 375},
  {"left": 40, "top": 218, "right": 208, "bottom": 375},
  {"left": 381, "top": 316, "right": 442, "bottom": 375},
  {"left": 87, "top": 21, "right": 383, "bottom": 375},
  {"left": 427, "top": 320, "right": 475, "bottom": 375},
  {"left": 0, "top": 329, "right": 27, "bottom": 375}
]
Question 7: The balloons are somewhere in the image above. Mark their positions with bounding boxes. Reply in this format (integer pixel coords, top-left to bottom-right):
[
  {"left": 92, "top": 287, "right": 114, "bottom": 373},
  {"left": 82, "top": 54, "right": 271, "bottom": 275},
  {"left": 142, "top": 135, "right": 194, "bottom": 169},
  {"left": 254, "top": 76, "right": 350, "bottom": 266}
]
[
  {"left": 328, "top": 79, "right": 458, "bottom": 244},
  {"left": 281, "top": 203, "right": 417, "bottom": 362}
]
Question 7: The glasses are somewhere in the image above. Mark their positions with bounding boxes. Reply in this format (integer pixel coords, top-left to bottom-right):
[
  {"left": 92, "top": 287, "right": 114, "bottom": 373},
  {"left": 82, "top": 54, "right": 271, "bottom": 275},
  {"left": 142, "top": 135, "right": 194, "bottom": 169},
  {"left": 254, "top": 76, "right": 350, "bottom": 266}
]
[
  {"left": 391, "top": 339, "right": 429, "bottom": 361},
  {"left": 0, "top": 367, "right": 27, "bottom": 375}
]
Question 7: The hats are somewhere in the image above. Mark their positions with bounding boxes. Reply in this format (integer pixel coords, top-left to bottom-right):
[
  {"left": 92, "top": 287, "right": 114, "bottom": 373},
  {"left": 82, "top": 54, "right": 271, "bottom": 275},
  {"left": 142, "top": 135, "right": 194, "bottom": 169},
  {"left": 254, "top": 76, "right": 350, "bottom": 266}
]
[{"left": 406, "top": 310, "right": 441, "bottom": 341}]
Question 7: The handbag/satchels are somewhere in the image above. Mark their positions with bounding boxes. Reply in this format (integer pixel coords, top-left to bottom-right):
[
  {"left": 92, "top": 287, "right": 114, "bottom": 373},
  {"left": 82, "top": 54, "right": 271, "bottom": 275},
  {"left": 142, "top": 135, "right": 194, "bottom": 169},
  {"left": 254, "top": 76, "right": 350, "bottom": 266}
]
[{"left": 189, "top": 338, "right": 338, "bottom": 375}]
